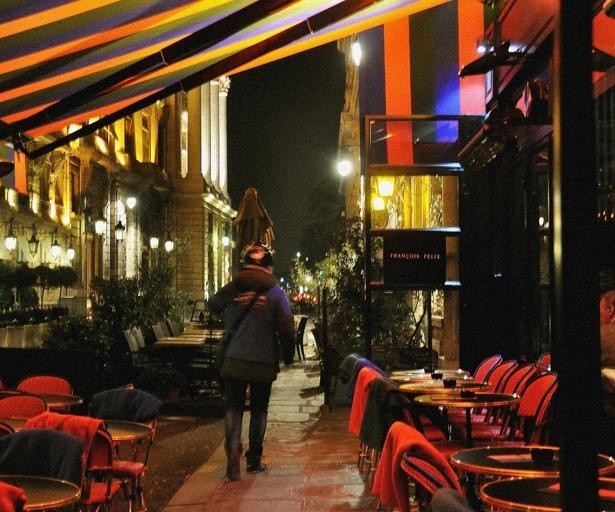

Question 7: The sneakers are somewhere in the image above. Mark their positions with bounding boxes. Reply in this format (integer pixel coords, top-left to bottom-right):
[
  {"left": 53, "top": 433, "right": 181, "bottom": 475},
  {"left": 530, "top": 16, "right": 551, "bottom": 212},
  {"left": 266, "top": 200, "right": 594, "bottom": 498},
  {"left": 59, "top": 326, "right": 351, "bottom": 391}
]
[
  {"left": 245, "top": 460, "right": 267, "bottom": 474},
  {"left": 226, "top": 462, "right": 242, "bottom": 479}
]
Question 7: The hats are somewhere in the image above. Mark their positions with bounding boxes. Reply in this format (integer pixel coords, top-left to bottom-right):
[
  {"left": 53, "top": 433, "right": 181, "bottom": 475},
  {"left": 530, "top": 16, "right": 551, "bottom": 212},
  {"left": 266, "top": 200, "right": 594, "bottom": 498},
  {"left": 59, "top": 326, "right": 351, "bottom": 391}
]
[{"left": 239, "top": 240, "right": 276, "bottom": 274}]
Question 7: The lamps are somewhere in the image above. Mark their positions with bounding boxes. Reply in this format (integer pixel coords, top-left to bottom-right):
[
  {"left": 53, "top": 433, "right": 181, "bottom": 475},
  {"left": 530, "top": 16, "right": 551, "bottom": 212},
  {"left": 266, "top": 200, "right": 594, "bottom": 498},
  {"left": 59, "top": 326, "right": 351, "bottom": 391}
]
[{"left": 5, "top": 182, "right": 174, "bottom": 260}]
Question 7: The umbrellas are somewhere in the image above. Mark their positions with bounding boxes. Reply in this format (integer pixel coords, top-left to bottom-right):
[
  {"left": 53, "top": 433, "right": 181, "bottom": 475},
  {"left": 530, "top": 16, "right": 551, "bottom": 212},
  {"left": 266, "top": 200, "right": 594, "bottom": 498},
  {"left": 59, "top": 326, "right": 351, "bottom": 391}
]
[{"left": 233, "top": 187, "right": 275, "bottom": 246}]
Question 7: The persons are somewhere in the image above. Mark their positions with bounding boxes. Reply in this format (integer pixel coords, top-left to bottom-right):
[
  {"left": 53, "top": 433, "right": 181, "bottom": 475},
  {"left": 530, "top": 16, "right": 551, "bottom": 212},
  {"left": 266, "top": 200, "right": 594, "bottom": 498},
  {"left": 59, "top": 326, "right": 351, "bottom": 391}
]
[{"left": 206, "top": 245, "right": 293, "bottom": 480}]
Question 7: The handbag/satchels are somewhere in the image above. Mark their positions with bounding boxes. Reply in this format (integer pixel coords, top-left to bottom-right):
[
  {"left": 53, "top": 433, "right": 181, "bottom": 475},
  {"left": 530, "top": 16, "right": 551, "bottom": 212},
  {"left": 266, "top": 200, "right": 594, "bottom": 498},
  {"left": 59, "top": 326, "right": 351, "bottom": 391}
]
[{"left": 215, "top": 344, "right": 228, "bottom": 394}]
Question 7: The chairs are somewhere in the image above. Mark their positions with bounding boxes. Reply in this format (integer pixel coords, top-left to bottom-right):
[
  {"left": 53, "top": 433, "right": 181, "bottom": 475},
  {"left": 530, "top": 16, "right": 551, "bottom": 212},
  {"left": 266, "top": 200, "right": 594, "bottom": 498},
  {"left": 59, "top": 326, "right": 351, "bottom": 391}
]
[{"left": 293, "top": 316, "right": 309, "bottom": 361}]
